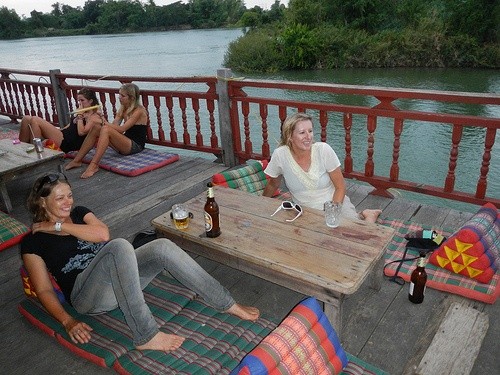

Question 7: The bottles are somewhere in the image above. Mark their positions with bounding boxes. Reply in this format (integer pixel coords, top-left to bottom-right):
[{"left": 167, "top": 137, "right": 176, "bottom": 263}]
[
  {"left": 408, "top": 252, "right": 428, "bottom": 304},
  {"left": 204, "top": 182, "right": 220, "bottom": 238}
]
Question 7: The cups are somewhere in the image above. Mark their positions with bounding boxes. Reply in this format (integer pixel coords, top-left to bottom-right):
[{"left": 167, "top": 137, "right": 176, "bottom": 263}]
[
  {"left": 172, "top": 203, "right": 189, "bottom": 229},
  {"left": 324, "top": 200, "right": 342, "bottom": 228}
]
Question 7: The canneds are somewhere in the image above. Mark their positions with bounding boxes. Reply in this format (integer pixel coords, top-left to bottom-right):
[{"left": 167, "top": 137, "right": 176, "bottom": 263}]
[{"left": 33, "top": 138, "right": 43, "bottom": 153}]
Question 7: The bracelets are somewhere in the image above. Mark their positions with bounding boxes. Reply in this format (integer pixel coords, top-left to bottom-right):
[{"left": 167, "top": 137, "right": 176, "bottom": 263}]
[
  {"left": 76, "top": 114, "right": 84, "bottom": 120},
  {"left": 55, "top": 221, "right": 63, "bottom": 231}
]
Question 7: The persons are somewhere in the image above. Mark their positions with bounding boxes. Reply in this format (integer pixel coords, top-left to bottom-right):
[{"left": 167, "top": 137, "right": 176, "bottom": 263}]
[
  {"left": 65, "top": 84, "right": 147, "bottom": 178},
  {"left": 19, "top": 88, "right": 102, "bottom": 153},
  {"left": 19, "top": 172, "right": 259, "bottom": 351},
  {"left": 263, "top": 114, "right": 382, "bottom": 222}
]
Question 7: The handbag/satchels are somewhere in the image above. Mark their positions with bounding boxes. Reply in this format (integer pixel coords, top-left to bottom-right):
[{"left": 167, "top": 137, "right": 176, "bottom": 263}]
[{"left": 404, "top": 229, "right": 446, "bottom": 250}]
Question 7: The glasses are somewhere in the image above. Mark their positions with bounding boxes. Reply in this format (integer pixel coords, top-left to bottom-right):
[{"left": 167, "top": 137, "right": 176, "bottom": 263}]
[
  {"left": 270, "top": 200, "right": 302, "bottom": 222},
  {"left": 35, "top": 173, "right": 68, "bottom": 195}
]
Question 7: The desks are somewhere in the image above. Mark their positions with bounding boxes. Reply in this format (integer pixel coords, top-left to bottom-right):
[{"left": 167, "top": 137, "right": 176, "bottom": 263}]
[
  {"left": 0, "top": 138, "right": 65, "bottom": 212},
  {"left": 151, "top": 185, "right": 396, "bottom": 347}
]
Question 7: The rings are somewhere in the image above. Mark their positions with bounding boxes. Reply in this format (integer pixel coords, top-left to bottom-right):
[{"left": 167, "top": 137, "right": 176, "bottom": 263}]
[{"left": 77, "top": 329, "right": 80, "bottom": 333}]
